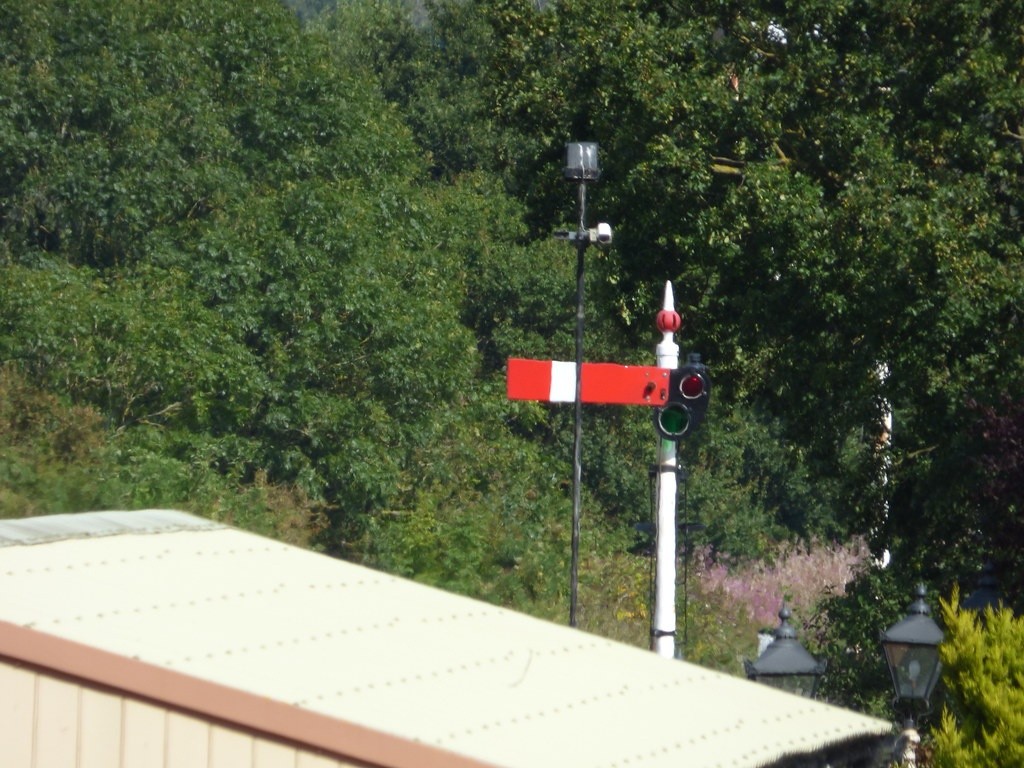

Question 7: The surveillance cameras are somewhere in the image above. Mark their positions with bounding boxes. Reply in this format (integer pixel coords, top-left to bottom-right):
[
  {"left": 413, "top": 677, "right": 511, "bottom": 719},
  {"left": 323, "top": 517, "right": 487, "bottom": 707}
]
[{"left": 596, "top": 222, "right": 613, "bottom": 244}]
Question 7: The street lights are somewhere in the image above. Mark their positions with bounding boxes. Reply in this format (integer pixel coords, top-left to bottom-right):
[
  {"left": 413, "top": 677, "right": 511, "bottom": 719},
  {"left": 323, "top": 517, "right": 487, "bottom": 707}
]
[
  {"left": 878, "top": 582, "right": 945, "bottom": 768},
  {"left": 563, "top": 138, "right": 601, "bottom": 626}
]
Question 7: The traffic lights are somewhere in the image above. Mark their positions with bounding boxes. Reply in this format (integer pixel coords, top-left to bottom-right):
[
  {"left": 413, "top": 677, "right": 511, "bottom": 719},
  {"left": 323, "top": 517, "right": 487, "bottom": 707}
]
[{"left": 654, "top": 369, "right": 708, "bottom": 442}]
